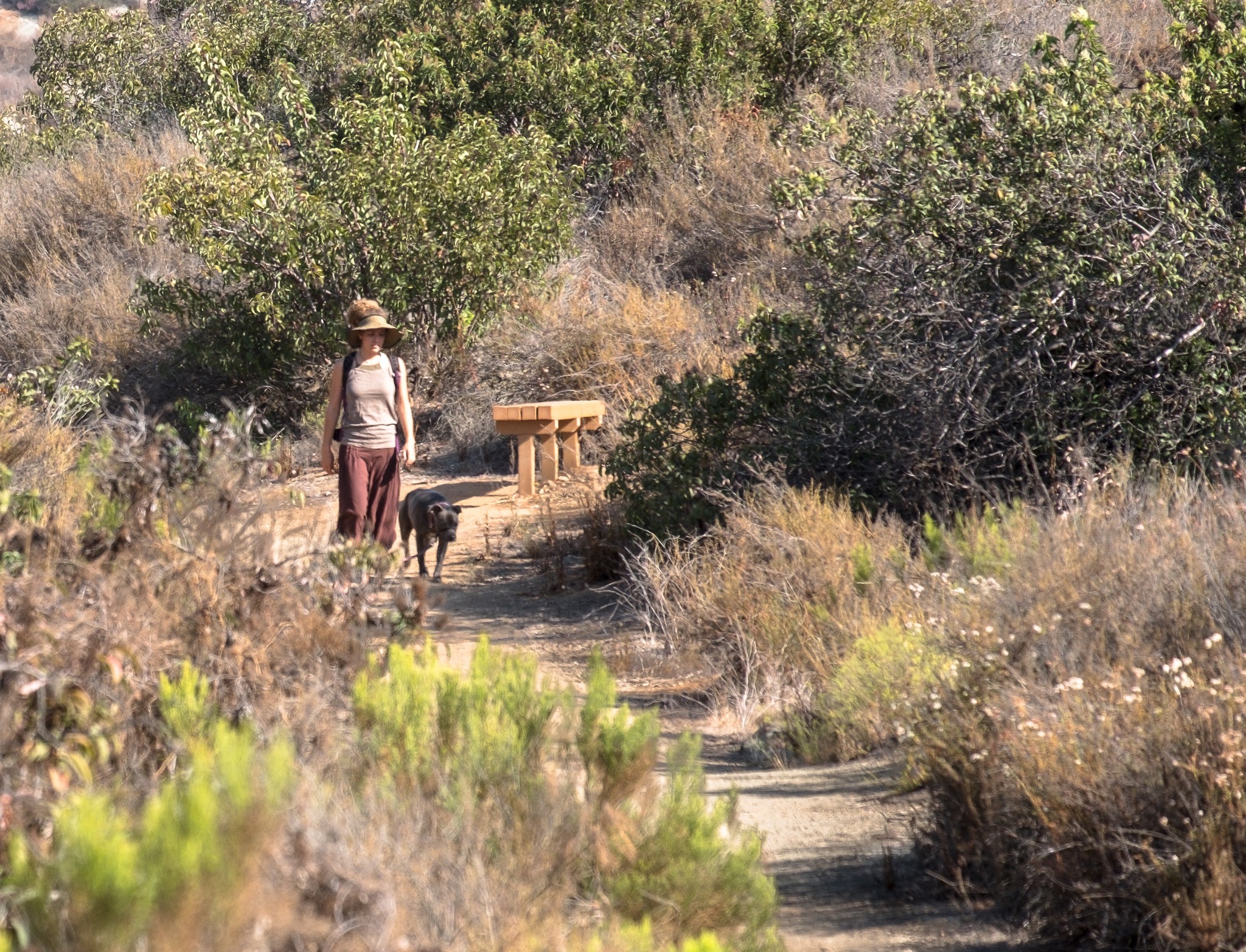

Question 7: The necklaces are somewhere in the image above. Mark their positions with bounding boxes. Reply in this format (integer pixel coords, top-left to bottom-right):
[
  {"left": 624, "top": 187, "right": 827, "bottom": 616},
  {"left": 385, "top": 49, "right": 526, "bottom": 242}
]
[{"left": 359, "top": 350, "right": 375, "bottom": 367}]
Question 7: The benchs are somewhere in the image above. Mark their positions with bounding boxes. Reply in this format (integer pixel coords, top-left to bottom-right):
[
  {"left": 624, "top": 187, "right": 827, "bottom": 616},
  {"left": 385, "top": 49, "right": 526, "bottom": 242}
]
[{"left": 492, "top": 401, "right": 605, "bottom": 498}]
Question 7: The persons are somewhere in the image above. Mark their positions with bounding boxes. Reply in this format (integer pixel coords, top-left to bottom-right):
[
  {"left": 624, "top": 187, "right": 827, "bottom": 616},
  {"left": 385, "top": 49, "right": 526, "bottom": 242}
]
[{"left": 318, "top": 300, "right": 415, "bottom": 584}]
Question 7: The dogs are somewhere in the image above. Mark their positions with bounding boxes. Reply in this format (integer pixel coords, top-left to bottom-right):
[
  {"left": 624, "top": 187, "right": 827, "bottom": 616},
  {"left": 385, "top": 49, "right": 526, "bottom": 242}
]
[{"left": 398, "top": 487, "right": 462, "bottom": 582}]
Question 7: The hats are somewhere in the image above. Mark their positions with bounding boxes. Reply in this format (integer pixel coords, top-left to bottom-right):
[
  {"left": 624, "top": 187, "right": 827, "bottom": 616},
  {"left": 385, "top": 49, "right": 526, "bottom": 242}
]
[{"left": 346, "top": 314, "right": 403, "bottom": 348}]
